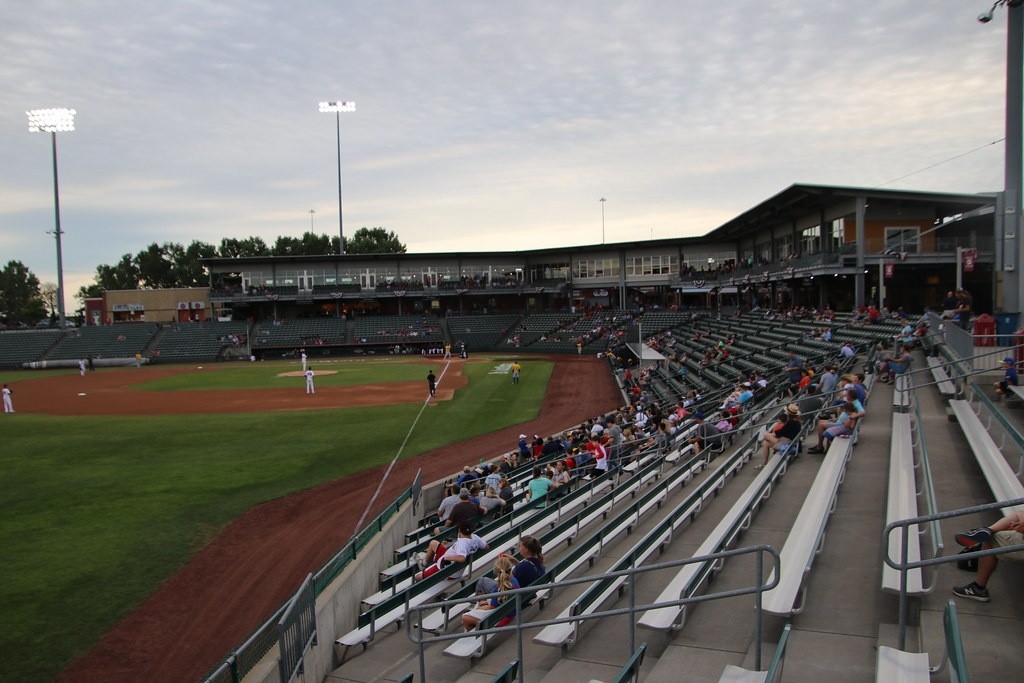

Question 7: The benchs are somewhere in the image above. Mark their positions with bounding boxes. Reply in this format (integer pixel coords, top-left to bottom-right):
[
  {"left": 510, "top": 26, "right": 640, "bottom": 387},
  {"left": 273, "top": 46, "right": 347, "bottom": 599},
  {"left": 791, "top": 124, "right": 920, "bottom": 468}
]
[{"left": 333, "top": 308, "right": 1024, "bottom": 683}]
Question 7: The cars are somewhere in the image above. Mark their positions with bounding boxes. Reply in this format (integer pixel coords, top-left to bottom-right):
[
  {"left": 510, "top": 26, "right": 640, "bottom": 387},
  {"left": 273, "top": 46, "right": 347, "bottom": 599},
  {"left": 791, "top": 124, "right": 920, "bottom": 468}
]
[{"left": 36, "top": 319, "right": 79, "bottom": 328}]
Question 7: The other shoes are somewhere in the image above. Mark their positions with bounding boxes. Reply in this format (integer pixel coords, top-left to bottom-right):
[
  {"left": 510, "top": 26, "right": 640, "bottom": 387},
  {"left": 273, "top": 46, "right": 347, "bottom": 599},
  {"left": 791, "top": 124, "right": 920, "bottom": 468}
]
[
  {"left": 444, "top": 480, "right": 448, "bottom": 488},
  {"left": 1004, "top": 393, "right": 1015, "bottom": 399},
  {"left": 754, "top": 464, "right": 765, "bottom": 469},
  {"left": 807, "top": 445, "right": 825, "bottom": 454},
  {"left": 449, "top": 479, "right": 453, "bottom": 484}
]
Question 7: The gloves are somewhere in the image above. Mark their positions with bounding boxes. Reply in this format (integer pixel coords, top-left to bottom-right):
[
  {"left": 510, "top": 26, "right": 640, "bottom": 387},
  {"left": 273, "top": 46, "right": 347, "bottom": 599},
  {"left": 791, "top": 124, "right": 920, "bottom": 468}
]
[{"left": 440, "top": 558, "right": 451, "bottom": 569}]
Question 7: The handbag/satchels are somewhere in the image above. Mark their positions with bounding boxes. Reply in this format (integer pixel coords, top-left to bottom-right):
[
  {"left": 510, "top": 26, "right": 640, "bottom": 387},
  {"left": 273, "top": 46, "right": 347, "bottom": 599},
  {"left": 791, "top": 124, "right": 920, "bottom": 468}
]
[{"left": 957, "top": 543, "right": 982, "bottom": 572}]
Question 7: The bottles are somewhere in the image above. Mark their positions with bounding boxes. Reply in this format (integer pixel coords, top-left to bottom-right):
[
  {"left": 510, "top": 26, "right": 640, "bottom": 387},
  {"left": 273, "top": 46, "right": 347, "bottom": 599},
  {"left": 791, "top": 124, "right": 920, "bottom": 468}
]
[{"left": 388, "top": 562, "right": 392, "bottom": 568}]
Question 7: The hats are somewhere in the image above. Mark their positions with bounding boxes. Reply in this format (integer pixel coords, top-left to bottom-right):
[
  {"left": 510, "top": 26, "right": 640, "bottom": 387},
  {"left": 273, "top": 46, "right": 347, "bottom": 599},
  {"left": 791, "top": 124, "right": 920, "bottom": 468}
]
[
  {"left": 463, "top": 465, "right": 470, "bottom": 471},
  {"left": 460, "top": 488, "right": 471, "bottom": 497},
  {"left": 503, "top": 453, "right": 510, "bottom": 459},
  {"left": 997, "top": 356, "right": 1014, "bottom": 363},
  {"left": 533, "top": 434, "right": 539, "bottom": 438},
  {"left": 482, "top": 462, "right": 493, "bottom": 468},
  {"left": 784, "top": 403, "right": 801, "bottom": 415},
  {"left": 694, "top": 413, "right": 705, "bottom": 418},
  {"left": 519, "top": 434, "right": 527, "bottom": 438}
]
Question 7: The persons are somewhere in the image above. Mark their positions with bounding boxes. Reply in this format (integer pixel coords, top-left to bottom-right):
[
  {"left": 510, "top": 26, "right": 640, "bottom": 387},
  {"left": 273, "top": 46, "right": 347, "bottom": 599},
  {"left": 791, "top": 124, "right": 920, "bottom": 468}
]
[
  {"left": 861, "top": 289, "right": 972, "bottom": 385},
  {"left": 273, "top": 303, "right": 470, "bottom": 359},
  {"left": 2, "top": 384, "right": 15, "bottom": 413},
  {"left": 195, "top": 313, "right": 199, "bottom": 322},
  {"left": 305, "top": 367, "right": 315, "bottom": 394},
  {"left": 415, "top": 434, "right": 608, "bottom": 633},
  {"left": 679, "top": 250, "right": 799, "bottom": 280},
  {"left": 498, "top": 324, "right": 561, "bottom": 349},
  {"left": 136, "top": 351, "right": 142, "bottom": 368},
  {"left": 217, "top": 333, "right": 247, "bottom": 362},
  {"left": 213, "top": 281, "right": 269, "bottom": 298},
  {"left": 480, "top": 305, "right": 487, "bottom": 316},
  {"left": 512, "top": 362, "right": 521, "bottom": 384},
  {"left": 427, "top": 370, "right": 436, "bottom": 397},
  {"left": 382, "top": 273, "right": 525, "bottom": 292},
  {"left": 561, "top": 295, "right": 871, "bottom": 472},
  {"left": 86, "top": 314, "right": 133, "bottom": 326},
  {"left": 952, "top": 509, "right": 1024, "bottom": 601},
  {"left": 994, "top": 356, "right": 1019, "bottom": 402},
  {"left": 79, "top": 353, "right": 100, "bottom": 376},
  {"left": 301, "top": 351, "right": 307, "bottom": 371}
]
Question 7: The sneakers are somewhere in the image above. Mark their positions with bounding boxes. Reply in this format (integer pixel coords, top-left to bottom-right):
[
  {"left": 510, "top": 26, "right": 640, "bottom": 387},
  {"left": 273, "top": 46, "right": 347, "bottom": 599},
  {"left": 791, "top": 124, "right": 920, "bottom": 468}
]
[
  {"left": 955, "top": 527, "right": 993, "bottom": 547},
  {"left": 413, "top": 552, "right": 425, "bottom": 570},
  {"left": 952, "top": 582, "right": 990, "bottom": 601}
]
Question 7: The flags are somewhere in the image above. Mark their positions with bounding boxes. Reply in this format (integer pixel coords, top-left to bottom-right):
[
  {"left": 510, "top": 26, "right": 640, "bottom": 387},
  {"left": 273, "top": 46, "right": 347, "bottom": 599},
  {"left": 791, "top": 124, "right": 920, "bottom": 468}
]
[
  {"left": 885, "top": 263, "right": 894, "bottom": 278},
  {"left": 964, "top": 250, "right": 975, "bottom": 272}
]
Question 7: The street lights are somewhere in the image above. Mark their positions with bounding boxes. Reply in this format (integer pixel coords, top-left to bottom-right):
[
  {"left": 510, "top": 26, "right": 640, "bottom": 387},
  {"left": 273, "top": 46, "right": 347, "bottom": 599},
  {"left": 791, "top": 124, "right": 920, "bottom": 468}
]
[
  {"left": 26, "top": 108, "right": 76, "bottom": 329},
  {"left": 600, "top": 198, "right": 606, "bottom": 244},
  {"left": 318, "top": 101, "right": 357, "bottom": 252}
]
